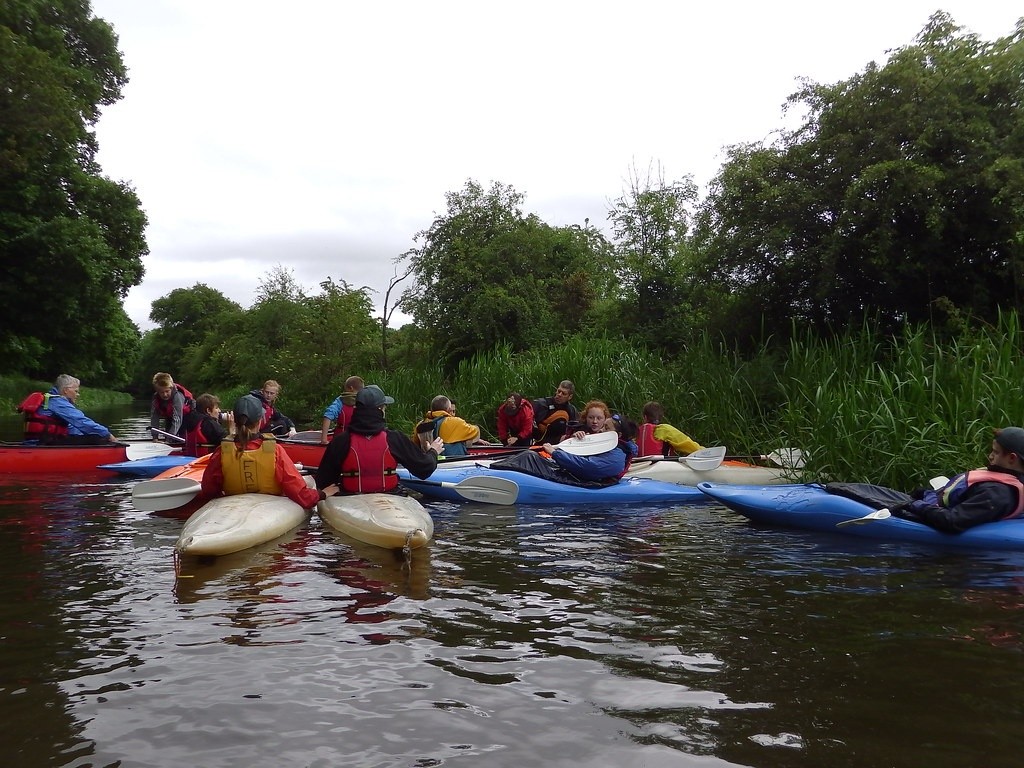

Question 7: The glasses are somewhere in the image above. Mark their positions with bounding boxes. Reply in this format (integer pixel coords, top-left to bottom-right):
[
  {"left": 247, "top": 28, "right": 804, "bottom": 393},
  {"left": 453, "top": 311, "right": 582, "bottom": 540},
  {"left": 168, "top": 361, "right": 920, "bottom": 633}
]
[
  {"left": 451, "top": 409, "right": 455, "bottom": 413},
  {"left": 612, "top": 414, "right": 623, "bottom": 429}
]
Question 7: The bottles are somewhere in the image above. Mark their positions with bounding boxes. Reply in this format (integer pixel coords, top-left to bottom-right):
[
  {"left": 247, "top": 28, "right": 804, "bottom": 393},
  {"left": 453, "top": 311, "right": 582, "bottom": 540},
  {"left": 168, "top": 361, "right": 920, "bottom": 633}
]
[{"left": 219, "top": 413, "right": 231, "bottom": 420}]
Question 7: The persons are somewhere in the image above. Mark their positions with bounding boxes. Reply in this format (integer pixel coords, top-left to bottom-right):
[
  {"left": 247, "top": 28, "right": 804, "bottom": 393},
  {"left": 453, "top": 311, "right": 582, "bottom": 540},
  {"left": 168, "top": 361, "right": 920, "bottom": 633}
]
[
  {"left": 412, "top": 394, "right": 490, "bottom": 456},
  {"left": 150, "top": 371, "right": 196, "bottom": 444},
  {"left": 249, "top": 380, "right": 298, "bottom": 439},
  {"left": 17, "top": 374, "right": 118, "bottom": 445},
  {"left": 184, "top": 392, "right": 235, "bottom": 457},
  {"left": 636, "top": 401, "right": 706, "bottom": 467},
  {"left": 319, "top": 376, "right": 365, "bottom": 446},
  {"left": 315, "top": 384, "right": 446, "bottom": 501},
  {"left": 496, "top": 393, "right": 534, "bottom": 447},
  {"left": 201, "top": 395, "right": 340, "bottom": 511},
  {"left": 530, "top": 380, "right": 578, "bottom": 446},
  {"left": 560, "top": 399, "right": 612, "bottom": 444},
  {"left": 489, "top": 413, "right": 639, "bottom": 489},
  {"left": 826, "top": 424, "right": 1024, "bottom": 534}
]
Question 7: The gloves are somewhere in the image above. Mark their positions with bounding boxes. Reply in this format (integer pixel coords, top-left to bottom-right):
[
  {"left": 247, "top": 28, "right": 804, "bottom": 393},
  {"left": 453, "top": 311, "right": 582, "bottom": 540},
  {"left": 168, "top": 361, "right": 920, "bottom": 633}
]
[
  {"left": 907, "top": 488, "right": 927, "bottom": 501},
  {"left": 890, "top": 499, "right": 915, "bottom": 514}
]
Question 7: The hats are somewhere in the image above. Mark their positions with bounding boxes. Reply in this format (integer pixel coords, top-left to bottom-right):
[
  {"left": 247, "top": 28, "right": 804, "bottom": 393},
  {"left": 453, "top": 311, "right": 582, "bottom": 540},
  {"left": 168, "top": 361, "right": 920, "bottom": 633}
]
[
  {"left": 233, "top": 395, "right": 266, "bottom": 424},
  {"left": 995, "top": 426, "right": 1024, "bottom": 461},
  {"left": 356, "top": 384, "right": 394, "bottom": 408},
  {"left": 506, "top": 393, "right": 521, "bottom": 414}
]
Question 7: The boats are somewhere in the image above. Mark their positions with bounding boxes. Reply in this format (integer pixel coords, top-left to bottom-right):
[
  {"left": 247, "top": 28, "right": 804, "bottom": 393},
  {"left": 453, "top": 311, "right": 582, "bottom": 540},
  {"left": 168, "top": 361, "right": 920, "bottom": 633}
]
[
  {"left": 621, "top": 458, "right": 830, "bottom": 485},
  {"left": 94, "top": 455, "right": 211, "bottom": 478},
  {"left": 175, "top": 473, "right": 320, "bottom": 557},
  {"left": 317, "top": 491, "right": 436, "bottom": 551},
  {"left": 394, "top": 458, "right": 719, "bottom": 504},
  {"left": 0, "top": 440, "right": 132, "bottom": 476},
  {"left": 696, "top": 480, "right": 1024, "bottom": 554},
  {"left": 167, "top": 411, "right": 637, "bottom": 475},
  {"left": 135, "top": 451, "right": 285, "bottom": 518}
]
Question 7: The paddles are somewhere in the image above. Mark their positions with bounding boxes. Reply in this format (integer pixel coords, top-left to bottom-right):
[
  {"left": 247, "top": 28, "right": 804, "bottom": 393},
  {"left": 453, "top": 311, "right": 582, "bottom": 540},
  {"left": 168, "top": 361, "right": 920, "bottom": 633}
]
[
  {"left": 437, "top": 430, "right": 618, "bottom": 464},
  {"left": 147, "top": 426, "right": 186, "bottom": 444},
  {"left": 294, "top": 463, "right": 519, "bottom": 506},
  {"left": 630, "top": 446, "right": 727, "bottom": 472},
  {"left": 633, "top": 448, "right": 811, "bottom": 469},
  {"left": 836, "top": 475, "right": 951, "bottom": 529},
  {"left": 109, "top": 440, "right": 129, "bottom": 446},
  {"left": 131, "top": 477, "right": 202, "bottom": 511},
  {"left": 125, "top": 431, "right": 323, "bottom": 461}
]
